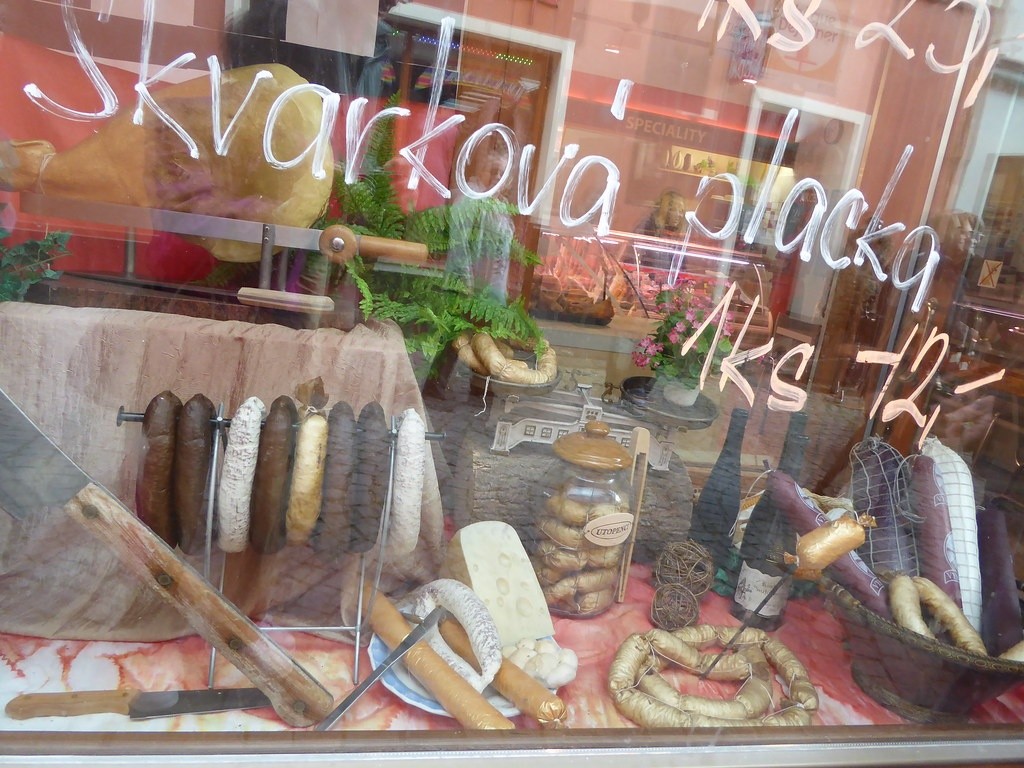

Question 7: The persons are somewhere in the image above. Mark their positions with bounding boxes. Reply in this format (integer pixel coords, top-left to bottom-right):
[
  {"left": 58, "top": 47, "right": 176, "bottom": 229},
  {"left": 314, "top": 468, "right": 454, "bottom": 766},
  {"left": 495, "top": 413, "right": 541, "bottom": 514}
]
[{"left": 422, "top": 151, "right": 516, "bottom": 409}]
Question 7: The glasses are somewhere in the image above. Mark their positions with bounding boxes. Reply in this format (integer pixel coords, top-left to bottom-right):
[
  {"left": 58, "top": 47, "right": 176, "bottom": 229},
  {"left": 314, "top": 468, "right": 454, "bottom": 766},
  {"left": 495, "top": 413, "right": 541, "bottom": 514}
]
[{"left": 482, "top": 169, "right": 505, "bottom": 177}]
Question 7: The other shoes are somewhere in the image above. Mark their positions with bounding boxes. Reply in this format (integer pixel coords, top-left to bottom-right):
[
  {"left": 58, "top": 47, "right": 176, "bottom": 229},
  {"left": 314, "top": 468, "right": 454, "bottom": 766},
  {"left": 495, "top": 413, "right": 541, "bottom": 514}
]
[
  {"left": 470, "top": 384, "right": 494, "bottom": 397},
  {"left": 422, "top": 377, "right": 456, "bottom": 402}
]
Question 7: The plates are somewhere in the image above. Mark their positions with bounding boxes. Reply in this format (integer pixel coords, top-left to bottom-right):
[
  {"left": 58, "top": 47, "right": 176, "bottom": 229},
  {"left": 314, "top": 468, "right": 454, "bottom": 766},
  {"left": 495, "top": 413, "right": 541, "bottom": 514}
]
[{"left": 367, "top": 604, "right": 561, "bottom": 718}]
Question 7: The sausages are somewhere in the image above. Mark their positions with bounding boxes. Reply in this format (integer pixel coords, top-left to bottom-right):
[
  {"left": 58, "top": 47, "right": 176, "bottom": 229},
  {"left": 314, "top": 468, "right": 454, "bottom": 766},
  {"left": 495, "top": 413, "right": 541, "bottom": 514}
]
[{"left": 131, "top": 328, "right": 1024, "bottom": 730}]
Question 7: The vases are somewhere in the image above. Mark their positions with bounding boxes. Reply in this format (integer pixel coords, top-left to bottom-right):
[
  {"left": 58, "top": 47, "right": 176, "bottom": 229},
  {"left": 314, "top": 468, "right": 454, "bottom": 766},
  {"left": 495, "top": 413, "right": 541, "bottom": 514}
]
[{"left": 663, "top": 378, "right": 701, "bottom": 405}]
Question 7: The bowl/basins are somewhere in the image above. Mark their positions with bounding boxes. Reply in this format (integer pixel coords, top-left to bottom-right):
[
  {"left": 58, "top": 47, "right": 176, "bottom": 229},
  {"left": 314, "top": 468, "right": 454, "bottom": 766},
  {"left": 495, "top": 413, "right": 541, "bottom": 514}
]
[{"left": 820, "top": 574, "right": 1024, "bottom": 724}]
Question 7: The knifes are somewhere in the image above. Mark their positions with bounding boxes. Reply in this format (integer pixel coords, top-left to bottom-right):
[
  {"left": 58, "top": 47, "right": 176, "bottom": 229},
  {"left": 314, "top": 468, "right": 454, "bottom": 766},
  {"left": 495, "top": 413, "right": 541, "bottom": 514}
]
[
  {"left": 0, "top": 390, "right": 332, "bottom": 727},
  {"left": 6, "top": 687, "right": 273, "bottom": 719},
  {"left": 53, "top": 268, "right": 335, "bottom": 314},
  {"left": 315, "top": 605, "right": 443, "bottom": 730}
]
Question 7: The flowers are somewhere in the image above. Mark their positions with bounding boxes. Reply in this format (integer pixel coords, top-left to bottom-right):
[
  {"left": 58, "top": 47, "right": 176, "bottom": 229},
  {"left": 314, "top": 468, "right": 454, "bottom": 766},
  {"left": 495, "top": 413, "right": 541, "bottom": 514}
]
[{"left": 633, "top": 279, "right": 766, "bottom": 389}]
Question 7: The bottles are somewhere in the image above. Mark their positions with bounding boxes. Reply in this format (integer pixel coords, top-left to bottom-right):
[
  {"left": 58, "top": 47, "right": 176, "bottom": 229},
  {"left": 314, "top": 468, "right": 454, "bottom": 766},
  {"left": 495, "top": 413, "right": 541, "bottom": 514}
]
[
  {"left": 735, "top": 412, "right": 808, "bottom": 577},
  {"left": 1003, "top": 239, "right": 1015, "bottom": 265},
  {"left": 686, "top": 406, "right": 748, "bottom": 578},
  {"left": 528, "top": 423, "right": 632, "bottom": 618},
  {"left": 731, "top": 436, "right": 808, "bottom": 633}
]
